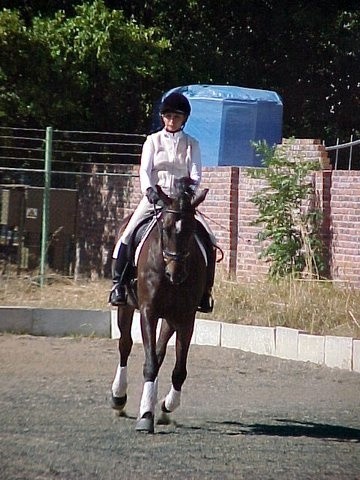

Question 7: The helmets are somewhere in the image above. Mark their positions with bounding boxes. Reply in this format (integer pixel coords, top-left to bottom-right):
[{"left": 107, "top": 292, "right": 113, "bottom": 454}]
[{"left": 160, "top": 93, "right": 190, "bottom": 116}]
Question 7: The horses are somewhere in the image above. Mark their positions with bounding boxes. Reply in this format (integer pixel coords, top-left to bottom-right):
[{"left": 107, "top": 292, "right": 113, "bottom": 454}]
[{"left": 111, "top": 184, "right": 209, "bottom": 434}]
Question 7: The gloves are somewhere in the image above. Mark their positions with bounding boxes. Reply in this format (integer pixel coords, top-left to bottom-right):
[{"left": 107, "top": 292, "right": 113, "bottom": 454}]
[
  {"left": 179, "top": 189, "right": 193, "bottom": 208},
  {"left": 146, "top": 188, "right": 159, "bottom": 203}
]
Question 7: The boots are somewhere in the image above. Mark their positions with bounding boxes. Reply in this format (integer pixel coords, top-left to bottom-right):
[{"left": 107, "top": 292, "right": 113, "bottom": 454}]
[
  {"left": 199, "top": 249, "right": 218, "bottom": 312},
  {"left": 113, "top": 243, "right": 128, "bottom": 302}
]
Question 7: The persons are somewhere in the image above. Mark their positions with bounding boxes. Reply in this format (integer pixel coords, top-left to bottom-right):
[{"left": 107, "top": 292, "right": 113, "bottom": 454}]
[{"left": 109, "top": 93, "right": 217, "bottom": 311}]
